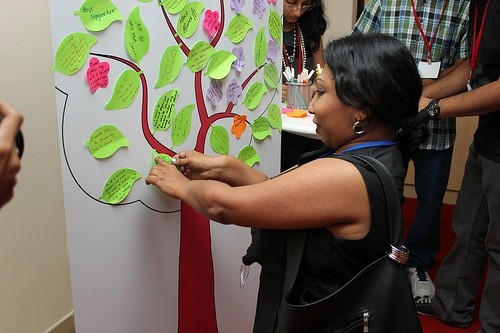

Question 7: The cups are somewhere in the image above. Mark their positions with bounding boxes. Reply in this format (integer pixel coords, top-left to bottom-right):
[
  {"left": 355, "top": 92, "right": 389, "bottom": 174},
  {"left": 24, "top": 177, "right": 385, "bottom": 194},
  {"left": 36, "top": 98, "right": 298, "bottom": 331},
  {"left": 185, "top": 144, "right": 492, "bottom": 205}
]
[{"left": 286, "top": 79, "right": 312, "bottom": 118}]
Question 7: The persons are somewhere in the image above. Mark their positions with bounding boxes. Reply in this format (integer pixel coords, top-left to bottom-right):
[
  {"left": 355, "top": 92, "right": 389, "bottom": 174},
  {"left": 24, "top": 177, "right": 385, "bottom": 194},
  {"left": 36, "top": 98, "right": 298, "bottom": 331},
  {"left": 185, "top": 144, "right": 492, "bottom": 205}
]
[
  {"left": 415, "top": 0, "right": 500, "bottom": 333},
  {"left": 146, "top": 32, "right": 423, "bottom": 333},
  {"left": 0, "top": 101, "right": 23, "bottom": 207},
  {"left": 279, "top": 0, "right": 327, "bottom": 102},
  {"left": 352, "top": 0, "right": 471, "bottom": 302}
]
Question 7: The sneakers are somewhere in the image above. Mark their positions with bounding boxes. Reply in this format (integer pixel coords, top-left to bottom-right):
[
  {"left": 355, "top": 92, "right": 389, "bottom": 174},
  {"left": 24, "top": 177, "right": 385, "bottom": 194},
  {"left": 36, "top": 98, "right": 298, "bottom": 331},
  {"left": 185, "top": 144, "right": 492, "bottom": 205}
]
[{"left": 406, "top": 267, "right": 436, "bottom": 300}]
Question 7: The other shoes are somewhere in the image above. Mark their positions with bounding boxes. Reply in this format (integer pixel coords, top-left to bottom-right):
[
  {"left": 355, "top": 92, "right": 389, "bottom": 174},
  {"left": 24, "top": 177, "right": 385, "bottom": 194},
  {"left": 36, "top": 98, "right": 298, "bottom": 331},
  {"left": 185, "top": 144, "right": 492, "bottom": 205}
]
[{"left": 417, "top": 304, "right": 472, "bottom": 328}]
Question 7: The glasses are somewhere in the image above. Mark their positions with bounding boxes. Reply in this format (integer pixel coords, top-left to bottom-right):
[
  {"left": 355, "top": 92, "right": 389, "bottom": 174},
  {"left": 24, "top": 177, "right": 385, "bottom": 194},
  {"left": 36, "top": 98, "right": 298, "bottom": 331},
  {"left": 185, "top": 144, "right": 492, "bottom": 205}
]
[{"left": 284, "top": 0, "right": 315, "bottom": 10}]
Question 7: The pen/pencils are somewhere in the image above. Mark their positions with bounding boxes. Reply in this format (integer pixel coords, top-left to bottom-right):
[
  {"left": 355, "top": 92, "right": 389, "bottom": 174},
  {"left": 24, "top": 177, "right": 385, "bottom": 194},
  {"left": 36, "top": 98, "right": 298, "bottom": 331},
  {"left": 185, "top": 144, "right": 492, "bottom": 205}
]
[
  {"left": 282, "top": 80, "right": 288, "bottom": 86},
  {"left": 283, "top": 67, "right": 315, "bottom": 109}
]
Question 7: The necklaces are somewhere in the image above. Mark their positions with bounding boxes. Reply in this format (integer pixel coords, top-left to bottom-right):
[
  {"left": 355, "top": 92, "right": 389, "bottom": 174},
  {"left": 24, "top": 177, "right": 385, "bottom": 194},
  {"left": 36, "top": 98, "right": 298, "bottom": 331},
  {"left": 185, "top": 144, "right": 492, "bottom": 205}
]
[{"left": 282, "top": 22, "right": 307, "bottom": 71}]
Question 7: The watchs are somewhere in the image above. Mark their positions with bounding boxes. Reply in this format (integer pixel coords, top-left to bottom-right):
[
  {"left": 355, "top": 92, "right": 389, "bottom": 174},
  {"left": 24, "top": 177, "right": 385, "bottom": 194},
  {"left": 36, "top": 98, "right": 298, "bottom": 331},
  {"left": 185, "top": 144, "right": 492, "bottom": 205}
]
[{"left": 426, "top": 99, "right": 441, "bottom": 121}]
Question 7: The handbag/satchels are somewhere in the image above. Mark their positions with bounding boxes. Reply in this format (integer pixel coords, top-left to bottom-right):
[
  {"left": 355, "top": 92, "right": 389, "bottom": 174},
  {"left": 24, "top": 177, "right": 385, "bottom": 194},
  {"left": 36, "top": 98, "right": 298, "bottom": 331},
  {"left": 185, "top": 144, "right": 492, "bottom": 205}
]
[{"left": 277, "top": 154, "right": 422, "bottom": 333}]
